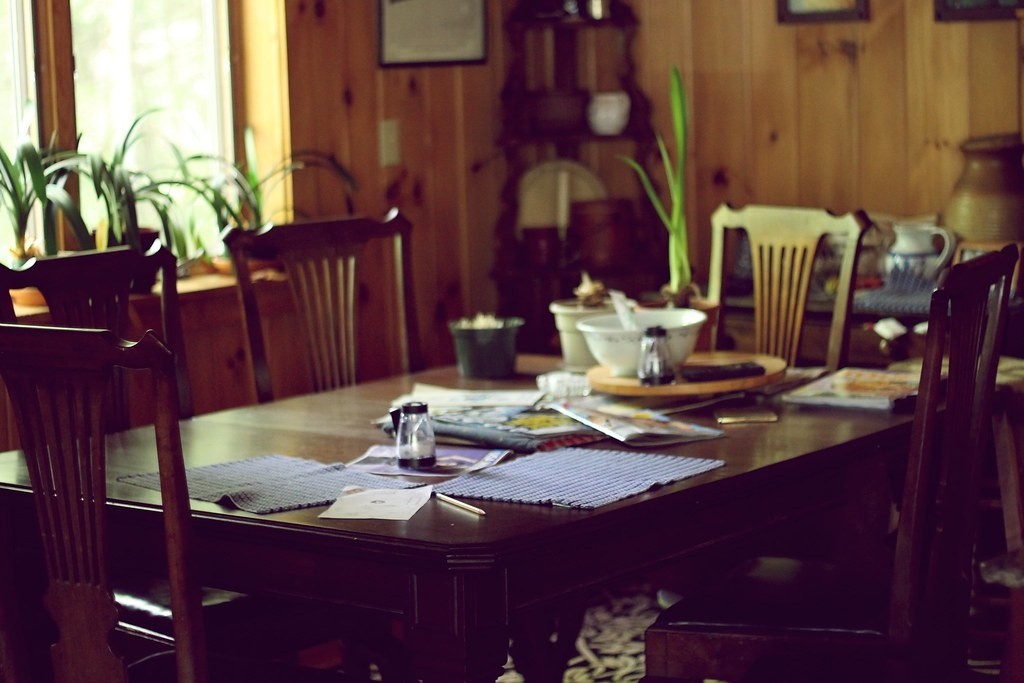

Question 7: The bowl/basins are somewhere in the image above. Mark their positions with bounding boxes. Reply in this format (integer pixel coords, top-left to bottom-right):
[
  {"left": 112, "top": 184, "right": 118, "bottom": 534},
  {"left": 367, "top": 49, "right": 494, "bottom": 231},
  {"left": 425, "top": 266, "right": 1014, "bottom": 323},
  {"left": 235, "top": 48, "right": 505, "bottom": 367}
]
[
  {"left": 447, "top": 319, "right": 524, "bottom": 380},
  {"left": 575, "top": 308, "right": 707, "bottom": 378},
  {"left": 521, "top": 89, "right": 587, "bottom": 136}
]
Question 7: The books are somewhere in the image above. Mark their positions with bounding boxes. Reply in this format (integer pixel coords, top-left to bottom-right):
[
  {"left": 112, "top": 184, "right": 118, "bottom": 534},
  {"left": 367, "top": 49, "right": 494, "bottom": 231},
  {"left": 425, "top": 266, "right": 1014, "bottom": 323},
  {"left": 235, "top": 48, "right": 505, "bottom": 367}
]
[
  {"left": 782, "top": 366, "right": 948, "bottom": 410},
  {"left": 371, "top": 382, "right": 727, "bottom": 446},
  {"left": 888, "top": 351, "right": 1024, "bottom": 385}
]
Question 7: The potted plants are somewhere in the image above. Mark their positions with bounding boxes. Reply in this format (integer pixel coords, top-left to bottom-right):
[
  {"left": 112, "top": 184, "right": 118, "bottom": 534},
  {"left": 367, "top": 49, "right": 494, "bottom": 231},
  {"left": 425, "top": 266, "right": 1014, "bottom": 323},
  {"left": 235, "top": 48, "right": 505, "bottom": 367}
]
[
  {"left": 549, "top": 271, "right": 630, "bottom": 375},
  {"left": 1, "top": 107, "right": 359, "bottom": 308},
  {"left": 613, "top": 64, "right": 720, "bottom": 354},
  {"left": 451, "top": 314, "right": 523, "bottom": 380}
]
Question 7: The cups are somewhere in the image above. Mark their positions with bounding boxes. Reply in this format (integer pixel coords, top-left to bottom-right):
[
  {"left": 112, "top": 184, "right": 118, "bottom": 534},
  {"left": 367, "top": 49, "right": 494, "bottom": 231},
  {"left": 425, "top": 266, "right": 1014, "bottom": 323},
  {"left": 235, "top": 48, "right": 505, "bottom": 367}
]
[
  {"left": 521, "top": 226, "right": 579, "bottom": 274},
  {"left": 588, "top": 89, "right": 632, "bottom": 139}
]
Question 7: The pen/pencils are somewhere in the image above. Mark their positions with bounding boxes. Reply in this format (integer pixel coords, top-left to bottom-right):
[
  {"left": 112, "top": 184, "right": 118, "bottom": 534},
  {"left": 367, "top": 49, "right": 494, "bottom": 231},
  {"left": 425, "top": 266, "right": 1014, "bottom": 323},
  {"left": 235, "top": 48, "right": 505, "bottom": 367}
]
[{"left": 436, "top": 492, "right": 487, "bottom": 515}]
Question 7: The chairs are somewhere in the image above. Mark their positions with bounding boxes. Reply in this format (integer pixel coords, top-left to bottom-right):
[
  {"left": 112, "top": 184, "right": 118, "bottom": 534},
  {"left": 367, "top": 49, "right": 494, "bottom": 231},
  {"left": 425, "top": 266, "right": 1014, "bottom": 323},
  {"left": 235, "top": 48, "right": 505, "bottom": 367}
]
[
  {"left": 708, "top": 203, "right": 871, "bottom": 372},
  {"left": 0, "top": 209, "right": 417, "bottom": 683},
  {"left": 614, "top": 246, "right": 1024, "bottom": 683}
]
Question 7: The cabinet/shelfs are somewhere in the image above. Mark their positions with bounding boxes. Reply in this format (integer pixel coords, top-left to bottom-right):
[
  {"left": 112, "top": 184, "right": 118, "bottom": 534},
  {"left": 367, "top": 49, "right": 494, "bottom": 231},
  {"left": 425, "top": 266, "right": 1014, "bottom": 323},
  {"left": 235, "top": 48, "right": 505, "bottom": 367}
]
[{"left": 487, "top": 0, "right": 671, "bottom": 355}]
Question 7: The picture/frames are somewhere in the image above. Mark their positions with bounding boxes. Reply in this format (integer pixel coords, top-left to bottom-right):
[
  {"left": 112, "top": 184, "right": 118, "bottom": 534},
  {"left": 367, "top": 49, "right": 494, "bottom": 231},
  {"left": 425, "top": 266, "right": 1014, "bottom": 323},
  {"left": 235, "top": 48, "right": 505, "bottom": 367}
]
[
  {"left": 778, "top": 0, "right": 871, "bottom": 25},
  {"left": 933, "top": 0, "right": 1018, "bottom": 22}
]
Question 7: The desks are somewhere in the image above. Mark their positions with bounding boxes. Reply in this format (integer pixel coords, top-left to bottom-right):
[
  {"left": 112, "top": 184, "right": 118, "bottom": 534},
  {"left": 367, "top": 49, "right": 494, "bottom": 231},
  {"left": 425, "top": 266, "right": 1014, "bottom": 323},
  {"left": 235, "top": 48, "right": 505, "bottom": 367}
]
[
  {"left": 635, "top": 284, "right": 1024, "bottom": 371},
  {"left": 1, "top": 349, "right": 1024, "bottom": 683}
]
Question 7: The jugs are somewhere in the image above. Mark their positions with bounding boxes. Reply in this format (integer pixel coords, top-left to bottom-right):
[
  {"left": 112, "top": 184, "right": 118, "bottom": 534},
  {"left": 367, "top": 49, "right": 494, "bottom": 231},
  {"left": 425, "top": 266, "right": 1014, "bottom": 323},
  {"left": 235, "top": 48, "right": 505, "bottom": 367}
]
[
  {"left": 569, "top": 199, "right": 650, "bottom": 273},
  {"left": 884, "top": 219, "right": 956, "bottom": 282}
]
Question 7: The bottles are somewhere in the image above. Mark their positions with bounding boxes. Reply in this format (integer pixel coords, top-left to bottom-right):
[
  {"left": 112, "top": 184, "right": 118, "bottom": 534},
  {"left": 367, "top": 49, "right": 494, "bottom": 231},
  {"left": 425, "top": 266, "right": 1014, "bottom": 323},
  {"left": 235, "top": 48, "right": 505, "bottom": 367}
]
[
  {"left": 939, "top": 133, "right": 1024, "bottom": 299},
  {"left": 397, "top": 401, "right": 436, "bottom": 469},
  {"left": 638, "top": 327, "right": 675, "bottom": 386}
]
